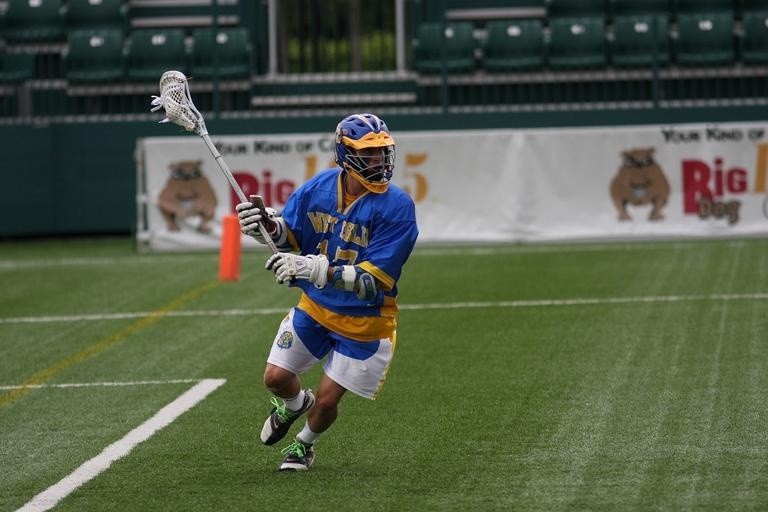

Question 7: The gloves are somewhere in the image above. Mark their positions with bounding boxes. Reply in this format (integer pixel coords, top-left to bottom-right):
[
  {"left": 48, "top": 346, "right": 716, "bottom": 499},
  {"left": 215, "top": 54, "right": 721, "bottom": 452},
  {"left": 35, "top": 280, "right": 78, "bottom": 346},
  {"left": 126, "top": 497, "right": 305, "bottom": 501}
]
[
  {"left": 265, "top": 247, "right": 331, "bottom": 290},
  {"left": 233, "top": 192, "right": 287, "bottom": 249}
]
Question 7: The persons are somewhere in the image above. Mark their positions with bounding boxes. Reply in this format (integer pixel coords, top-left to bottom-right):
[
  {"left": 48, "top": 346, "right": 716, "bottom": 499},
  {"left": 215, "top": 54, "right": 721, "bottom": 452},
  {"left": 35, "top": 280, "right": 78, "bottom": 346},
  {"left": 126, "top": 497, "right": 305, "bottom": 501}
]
[{"left": 235, "top": 112, "right": 419, "bottom": 471}]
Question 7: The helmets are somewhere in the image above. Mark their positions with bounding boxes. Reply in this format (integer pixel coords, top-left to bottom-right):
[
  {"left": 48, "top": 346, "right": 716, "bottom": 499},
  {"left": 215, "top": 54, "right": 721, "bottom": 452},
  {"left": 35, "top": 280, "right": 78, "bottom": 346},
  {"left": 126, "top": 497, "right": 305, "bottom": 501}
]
[{"left": 333, "top": 112, "right": 396, "bottom": 198}]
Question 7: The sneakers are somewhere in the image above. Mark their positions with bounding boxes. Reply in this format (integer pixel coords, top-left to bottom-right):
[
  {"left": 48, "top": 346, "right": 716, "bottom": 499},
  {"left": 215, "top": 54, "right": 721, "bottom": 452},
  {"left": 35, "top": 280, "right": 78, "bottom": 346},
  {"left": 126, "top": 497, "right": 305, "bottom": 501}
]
[
  {"left": 258, "top": 383, "right": 316, "bottom": 445},
  {"left": 275, "top": 436, "right": 319, "bottom": 473}
]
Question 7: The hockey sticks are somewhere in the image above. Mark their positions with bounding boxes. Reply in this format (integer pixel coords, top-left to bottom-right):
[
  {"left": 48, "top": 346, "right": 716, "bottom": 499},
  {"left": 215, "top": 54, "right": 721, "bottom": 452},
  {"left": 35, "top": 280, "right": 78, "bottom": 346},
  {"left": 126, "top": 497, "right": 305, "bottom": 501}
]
[{"left": 151, "top": 70, "right": 281, "bottom": 254}]
